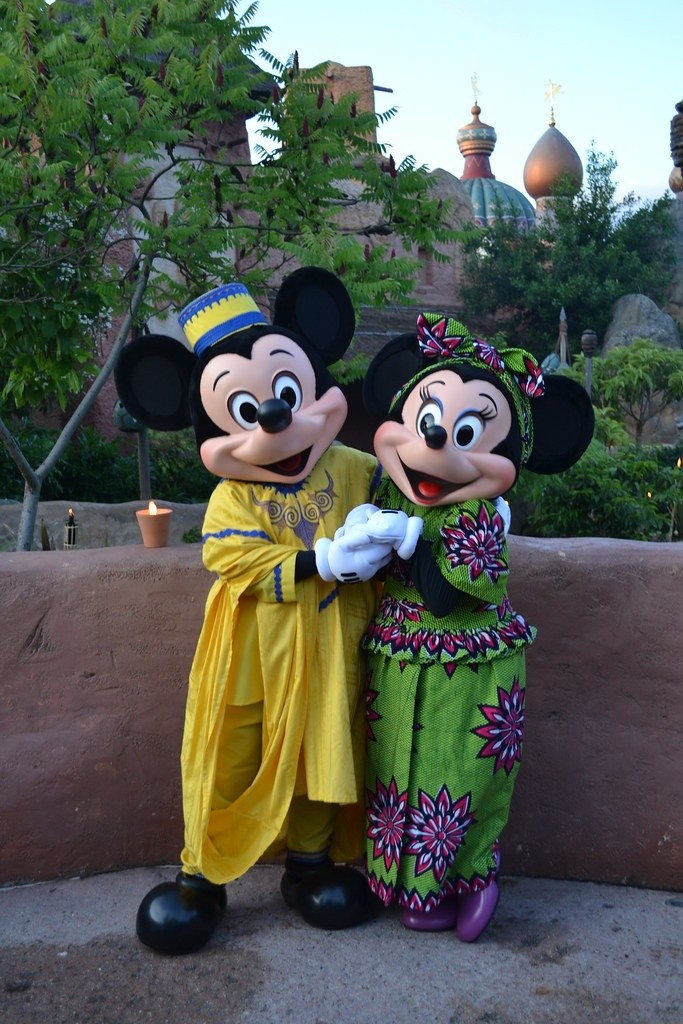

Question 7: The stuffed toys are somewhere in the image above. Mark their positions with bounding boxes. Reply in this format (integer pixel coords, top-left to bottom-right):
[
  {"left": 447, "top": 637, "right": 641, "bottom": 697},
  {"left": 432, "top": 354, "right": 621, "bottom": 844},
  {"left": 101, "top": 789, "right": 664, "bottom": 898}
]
[
  {"left": 333, "top": 313, "right": 595, "bottom": 942},
  {"left": 113, "top": 268, "right": 510, "bottom": 956}
]
